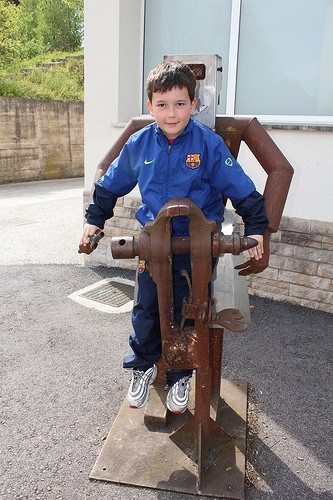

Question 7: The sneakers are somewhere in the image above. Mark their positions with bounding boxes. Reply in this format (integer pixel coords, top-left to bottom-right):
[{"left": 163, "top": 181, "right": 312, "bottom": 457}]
[{"left": 127, "top": 363, "right": 190, "bottom": 414}]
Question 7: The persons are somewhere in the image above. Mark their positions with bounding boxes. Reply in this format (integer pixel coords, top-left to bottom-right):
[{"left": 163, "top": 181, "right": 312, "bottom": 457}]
[{"left": 77, "top": 58, "right": 268, "bottom": 417}]
